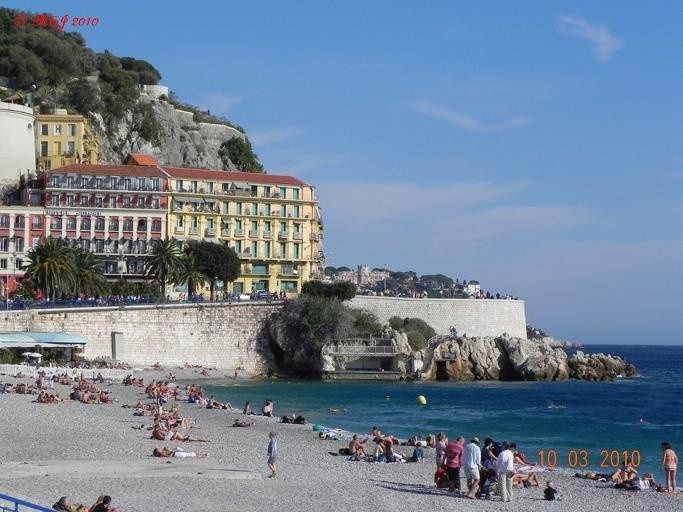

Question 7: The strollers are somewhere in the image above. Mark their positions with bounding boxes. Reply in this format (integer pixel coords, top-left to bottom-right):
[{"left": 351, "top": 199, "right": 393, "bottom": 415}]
[
  {"left": 475, "top": 463, "right": 501, "bottom": 501},
  {"left": 433, "top": 464, "right": 449, "bottom": 489}
]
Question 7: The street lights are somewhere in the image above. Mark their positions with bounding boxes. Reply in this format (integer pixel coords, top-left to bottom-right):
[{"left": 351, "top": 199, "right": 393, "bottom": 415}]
[
  {"left": 10, "top": 252, "right": 20, "bottom": 302},
  {"left": 116, "top": 250, "right": 128, "bottom": 282}
]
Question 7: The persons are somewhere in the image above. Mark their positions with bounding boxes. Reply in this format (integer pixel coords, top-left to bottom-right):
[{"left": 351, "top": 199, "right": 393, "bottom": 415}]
[
  {"left": 544, "top": 481, "right": 558, "bottom": 500},
  {"left": 0, "top": 361, "right": 231, "bottom": 458},
  {"left": 243, "top": 398, "right": 273, "bottom": 418},
  {"left": 267, "top": 432, "right": 278, "bottom": 477},
  {"left": 252, "top": 287, "right": 286, "bottom": 301},
  {"left": 280, "top": 415, "right": 306, "bottom": 424},
  {"left": 233, "top": 419, "right": 255, "bottom": 427},
  {"left": 178, "top": 290, "right": 242, "bottom": 304},
  {"left": 362, "top": 287, "right": 430, "bottom": 298},
  {"left": 11, "top": 295, "right": 146, "bottom": 309},
  {"left": 319, "top": 428, "right": 340, "bottom": 439},
  {"left": 53, "top": 495, "right": 117, "bottom": 512},
  {"left": 348, "top": 425, "right": 539, "bottom": 502},
  {"left": 475, "top": 290, "right": 517, "bottom": 300},
  {"left": 660, "top": 440, "right": 678, "bottom": 493},
  {"left": 575, "top": 457, "right": 656, "bottom": 491}
]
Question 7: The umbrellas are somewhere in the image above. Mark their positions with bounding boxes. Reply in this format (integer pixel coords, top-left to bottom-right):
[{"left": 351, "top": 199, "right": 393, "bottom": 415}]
[{"left": 22, "top": 352, "right": 43, "bottom": 359}]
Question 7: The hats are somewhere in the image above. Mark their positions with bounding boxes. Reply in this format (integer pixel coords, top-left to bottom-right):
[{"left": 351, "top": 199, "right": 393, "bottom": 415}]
[{"left": 471, "top": 437, "right": 481, "bottom": 446}]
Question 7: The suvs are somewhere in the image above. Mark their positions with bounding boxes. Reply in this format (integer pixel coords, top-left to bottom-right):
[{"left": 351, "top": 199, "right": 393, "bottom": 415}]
[{"left": 238, "top": 289, "right": 279, "bottom": 301}]
